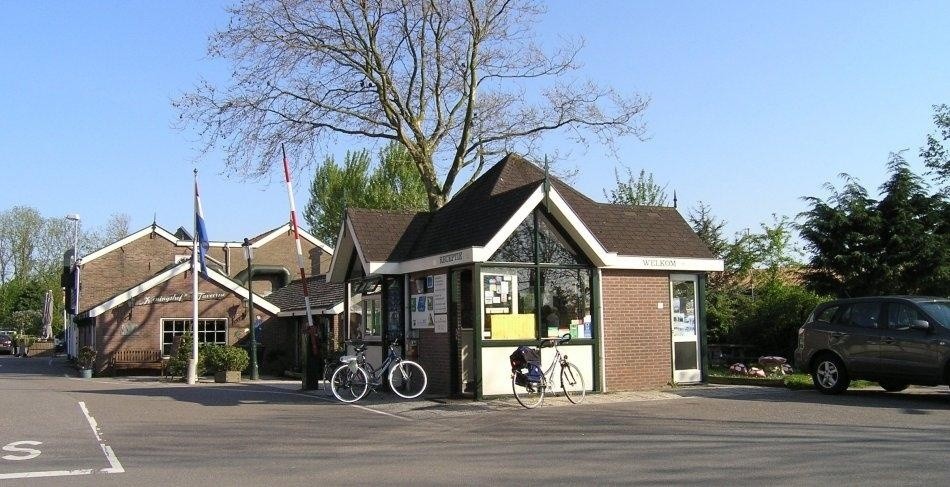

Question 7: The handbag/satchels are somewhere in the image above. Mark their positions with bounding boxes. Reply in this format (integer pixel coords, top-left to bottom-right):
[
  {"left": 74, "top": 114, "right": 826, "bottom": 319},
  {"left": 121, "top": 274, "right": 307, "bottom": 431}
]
[{"left": 509, "top": 344, "right": 543, "bottom": 385}]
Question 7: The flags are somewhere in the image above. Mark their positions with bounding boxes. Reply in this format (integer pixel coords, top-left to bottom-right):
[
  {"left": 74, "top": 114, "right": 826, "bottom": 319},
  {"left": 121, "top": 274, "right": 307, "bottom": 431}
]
[{"left": 195, "top": 180, "right": 209, "bottom": 280}]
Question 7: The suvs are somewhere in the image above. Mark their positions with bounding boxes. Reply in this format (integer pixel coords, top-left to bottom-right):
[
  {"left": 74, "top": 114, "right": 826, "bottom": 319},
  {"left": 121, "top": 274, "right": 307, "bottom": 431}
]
[{"left": 794, "top": 293, "right": 950, "bottom": 394}]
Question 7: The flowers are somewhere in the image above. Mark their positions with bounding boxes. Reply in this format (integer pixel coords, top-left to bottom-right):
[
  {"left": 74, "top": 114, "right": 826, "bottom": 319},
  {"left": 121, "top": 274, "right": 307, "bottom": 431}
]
[{"left": 78, "top": 344, "right": 99, "bottom": 369}]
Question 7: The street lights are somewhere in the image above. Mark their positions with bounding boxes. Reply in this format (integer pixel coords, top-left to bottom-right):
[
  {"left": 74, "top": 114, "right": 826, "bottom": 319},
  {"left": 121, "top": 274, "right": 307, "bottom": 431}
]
[
  {"left": 241, "top": 237, "right": 260, "bottom": 380},
  {"left": 65, "top": 213, "right": 80, "bottom": 264}
]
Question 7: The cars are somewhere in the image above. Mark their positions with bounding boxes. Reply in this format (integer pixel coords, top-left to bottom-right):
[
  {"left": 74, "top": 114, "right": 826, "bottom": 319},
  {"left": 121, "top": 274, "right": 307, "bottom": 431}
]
[{"left": 0, "top": 334, "right": 14, "bottom": 354}]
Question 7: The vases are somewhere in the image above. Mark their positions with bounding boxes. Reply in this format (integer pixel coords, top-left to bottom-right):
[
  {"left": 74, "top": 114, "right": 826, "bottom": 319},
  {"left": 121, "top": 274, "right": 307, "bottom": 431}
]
[{"left": 80, "top": 368, "right": 92, "bottom": 377}]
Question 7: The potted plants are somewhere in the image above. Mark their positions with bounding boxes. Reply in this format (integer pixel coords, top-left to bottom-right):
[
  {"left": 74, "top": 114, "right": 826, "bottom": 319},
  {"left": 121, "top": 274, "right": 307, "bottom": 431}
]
[{"left": 208, "top": 344, "right": 250, "bottom": 382}]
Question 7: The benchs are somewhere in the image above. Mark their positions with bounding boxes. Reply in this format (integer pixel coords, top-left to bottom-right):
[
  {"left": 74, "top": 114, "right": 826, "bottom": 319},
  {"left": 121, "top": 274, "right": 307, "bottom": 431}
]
[{"left": 107, "top": 348, "right": 168, "bottom": 377}]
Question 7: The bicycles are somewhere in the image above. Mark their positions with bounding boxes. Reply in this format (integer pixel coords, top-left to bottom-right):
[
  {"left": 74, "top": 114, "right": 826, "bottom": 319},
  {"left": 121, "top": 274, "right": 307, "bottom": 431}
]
[
  {"left": 512, "top": 332, "right": 586, "bottom": 408},
  {"left": 330, "top": 333, "right": 427, "bottom": 403},
  {"left": 323, "top": 343, "right": 375, "bottom": 398}
]
